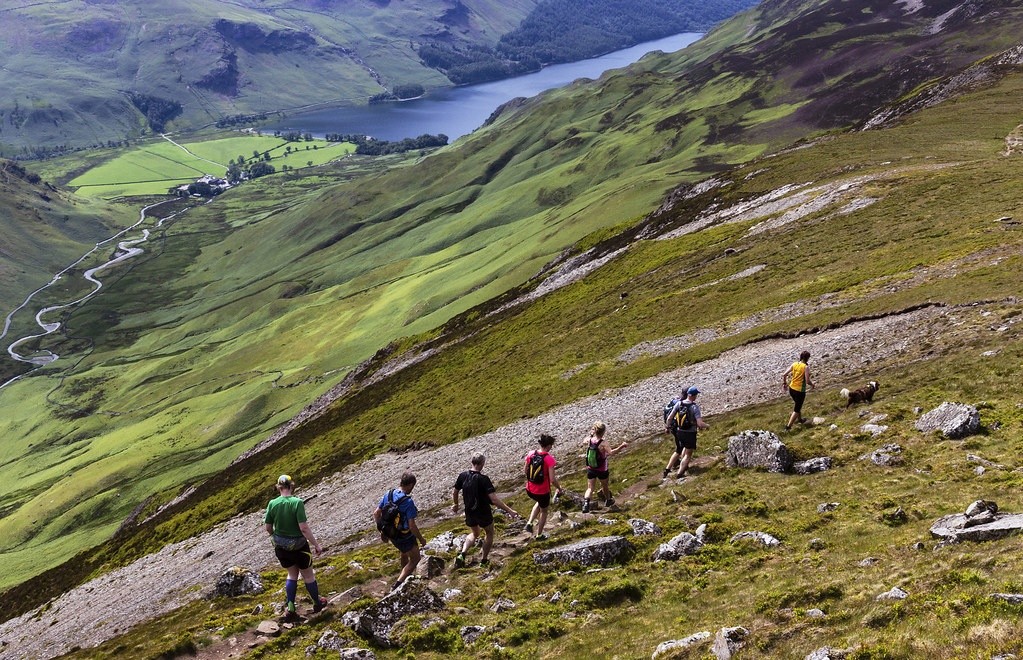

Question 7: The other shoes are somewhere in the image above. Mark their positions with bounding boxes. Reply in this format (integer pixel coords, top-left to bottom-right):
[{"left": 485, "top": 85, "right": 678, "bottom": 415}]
[
  {"left": 582, "top": 504, "right": 590, "bottom": 513},
  {"left": 314, "top": 597, "right": 327, "bottom": 614},
  {"left": 480, "top": 560, "right": 490, "bottom": 568},
  {"left": 673, "top": 459, "right": 681, "bottom": 468},
  {"left": 284, "top": 607, "right": 301, "bottom": 620},
  {"left": 535, "top": 534, "right": 548, "bottom": 541},
  {"left": 663, "top": 469, "right": 670, "bottom": 479},
  {"left": 456, "top": 554, "right": 465, "bottom": 569},
  {"left": 523, "top": 523, "right": 533, "bottom": 533},
  {"left": 605, "top": 499, "right": 616, "bottom": 507}
]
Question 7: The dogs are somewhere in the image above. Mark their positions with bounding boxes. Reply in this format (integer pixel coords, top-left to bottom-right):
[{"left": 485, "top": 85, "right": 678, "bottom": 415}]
[{"left": 839, "top": 380, "right": 881, "bottom": 411}]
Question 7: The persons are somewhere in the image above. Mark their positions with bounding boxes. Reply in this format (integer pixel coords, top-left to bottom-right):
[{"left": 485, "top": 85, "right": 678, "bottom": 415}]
[
  {"left": 264, "top": 475, "right": 328, "bottom": 619},
  {"left": 523, "top": 433, "right": 563, "bottom": 542},
  {"left": 666, "top": 385, "right": 711, "bottom": 478},
  {"left": 662, "top": 386, "right": 692, "bottom": 478},
  {"left": 373, "top": 471, "right": 427, "bottom": 594},
  {"left": 581, "top": 420, "right": 629, "bottom": 513},
  {"left": 782, "top": 351, "right": 816, "bottom": 432},
  {"left": 451, "top": 452, "right": 519, "bottom": 568}
]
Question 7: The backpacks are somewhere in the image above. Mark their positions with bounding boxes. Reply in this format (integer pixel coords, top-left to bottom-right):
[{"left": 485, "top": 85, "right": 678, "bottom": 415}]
[
  {"left": 665, "top": 400, "right": 676, "bottom": 426},
  {"left": 527, "top": 449, "right": 547, "bottom": 485},
  {"left": 585, "top": 438, "right": 606, "bottom": 468},
  {"left": 462, "top": 470, "right": 483, "bottom": 510},
  {"left": 676, "top": 401, "right": 696, "bottom": 430},
  {"left": 377, "top": 489, "right": 412, "bottom": 538}
]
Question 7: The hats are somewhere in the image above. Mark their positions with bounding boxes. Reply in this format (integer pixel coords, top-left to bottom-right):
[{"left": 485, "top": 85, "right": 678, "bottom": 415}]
[
  {"left": 682, "top": 386, "right": 700, "bottom": 395},
  {"left": 277, "top": 474, "right": 292, "bottom": 486}
]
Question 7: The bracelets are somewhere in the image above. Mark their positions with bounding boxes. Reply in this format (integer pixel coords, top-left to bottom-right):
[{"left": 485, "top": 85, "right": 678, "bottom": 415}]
[
  {"left": 589, "top": 434, "right": 593, "bottom": 437},
  {"left": 454, "top": 502, "right": 458, "bottom": 504},
  {"left": 783, "top": 383, "right": 787, "bottom": 385}
]
